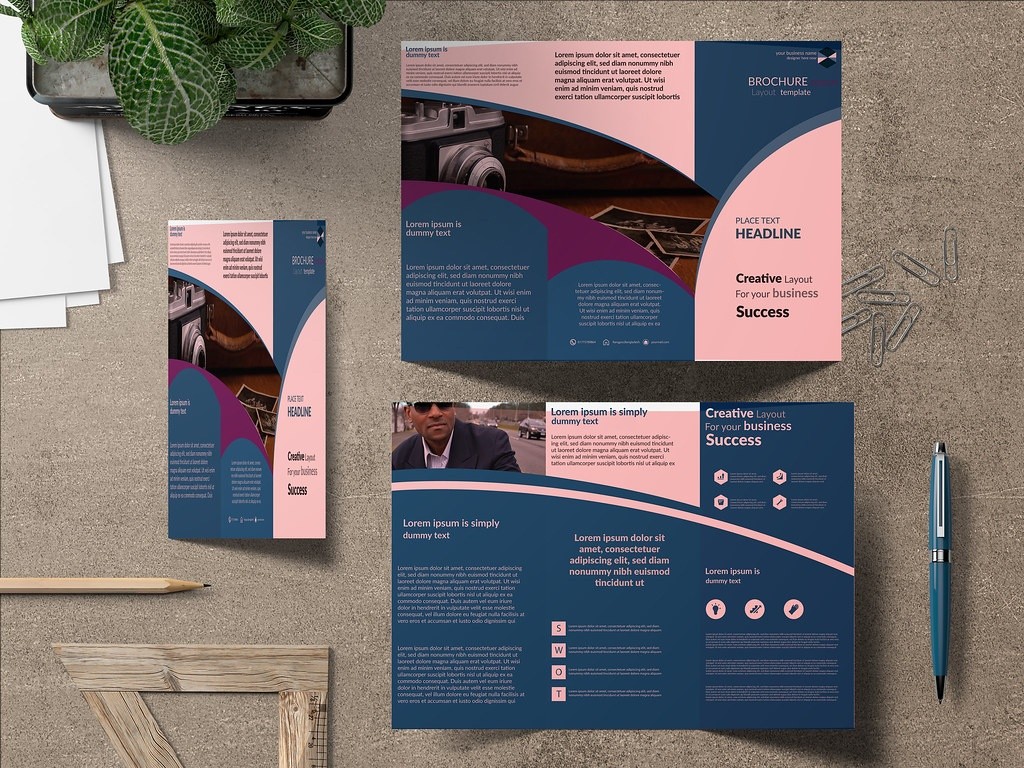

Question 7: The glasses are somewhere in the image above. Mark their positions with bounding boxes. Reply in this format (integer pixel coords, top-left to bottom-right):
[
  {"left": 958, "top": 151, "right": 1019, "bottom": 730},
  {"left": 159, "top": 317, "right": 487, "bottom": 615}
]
[{"left": 406, "top": 401, "right": 453, "bottom": 413}]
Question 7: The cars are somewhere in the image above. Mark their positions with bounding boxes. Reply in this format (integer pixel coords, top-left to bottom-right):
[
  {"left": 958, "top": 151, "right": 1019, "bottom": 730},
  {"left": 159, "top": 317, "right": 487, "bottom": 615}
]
[
  {"left": 486, "top": 419, "right": 498, "bottom": 428},
  {"left": 519, "top": 418, "right": 547, "bottom": 440}
]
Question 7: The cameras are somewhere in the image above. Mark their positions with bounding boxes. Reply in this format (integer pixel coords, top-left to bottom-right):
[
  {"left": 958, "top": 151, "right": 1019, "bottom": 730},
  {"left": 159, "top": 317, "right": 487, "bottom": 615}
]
[
  {"left": 400, "top": 100, "right": 510, "bottom": 195},
  {"left": 168, "top": 276, "right": 209, "bottom": 373}
]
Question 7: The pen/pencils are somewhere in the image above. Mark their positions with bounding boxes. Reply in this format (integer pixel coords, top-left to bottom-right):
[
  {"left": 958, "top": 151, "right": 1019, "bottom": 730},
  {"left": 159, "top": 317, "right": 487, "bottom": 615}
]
[
  {"left": 925, "top": 441, "right": 952, "bottom": 707},
  {"left": 0, "top": 576, "right": 212, "bottom": 598}
]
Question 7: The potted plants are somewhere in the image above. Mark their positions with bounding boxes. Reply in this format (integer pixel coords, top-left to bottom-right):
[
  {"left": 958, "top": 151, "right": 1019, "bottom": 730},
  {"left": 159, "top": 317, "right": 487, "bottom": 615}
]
[{"left": 0, "top": 0, "right": 387, "bottom": 145}]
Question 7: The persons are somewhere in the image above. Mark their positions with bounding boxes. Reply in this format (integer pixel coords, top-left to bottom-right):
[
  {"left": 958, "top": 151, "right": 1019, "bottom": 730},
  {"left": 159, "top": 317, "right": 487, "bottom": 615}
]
[{"left": 393, "top": 401, "right": 520, "bottom": 474}]
